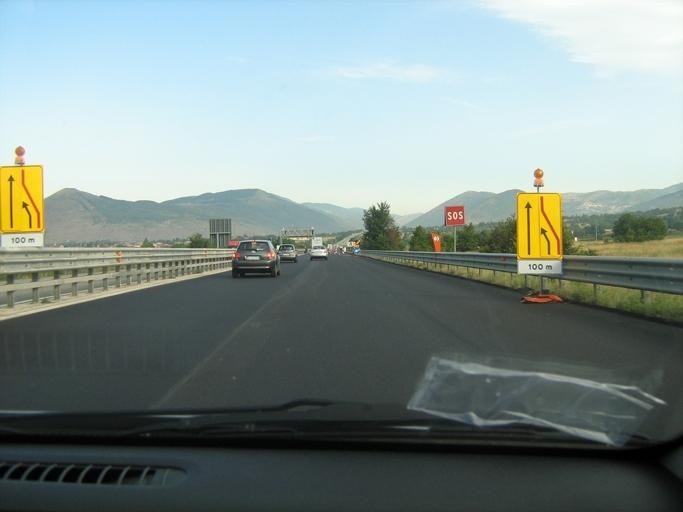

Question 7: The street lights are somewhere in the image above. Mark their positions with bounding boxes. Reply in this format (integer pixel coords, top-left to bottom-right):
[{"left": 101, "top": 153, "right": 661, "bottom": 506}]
[
  {"left": 12, "top": 144, "right": 24, "bottom": 166},
  {"left": 532, "top": 167, "right": 545, "bottom": 298}
]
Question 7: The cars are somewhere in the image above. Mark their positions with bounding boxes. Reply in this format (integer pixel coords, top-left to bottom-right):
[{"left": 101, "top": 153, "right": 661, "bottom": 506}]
[{"left": 309, "top": 244, "right": 327, "bottom": 261}]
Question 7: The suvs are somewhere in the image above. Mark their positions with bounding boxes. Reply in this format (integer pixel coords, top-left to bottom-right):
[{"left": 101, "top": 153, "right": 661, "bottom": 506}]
[
  {"left": 230, "top": 239, "right": 280, "bottom": 279},
  {"left": 277, "top": 244, "right": 297, "bottom": 263}
]
